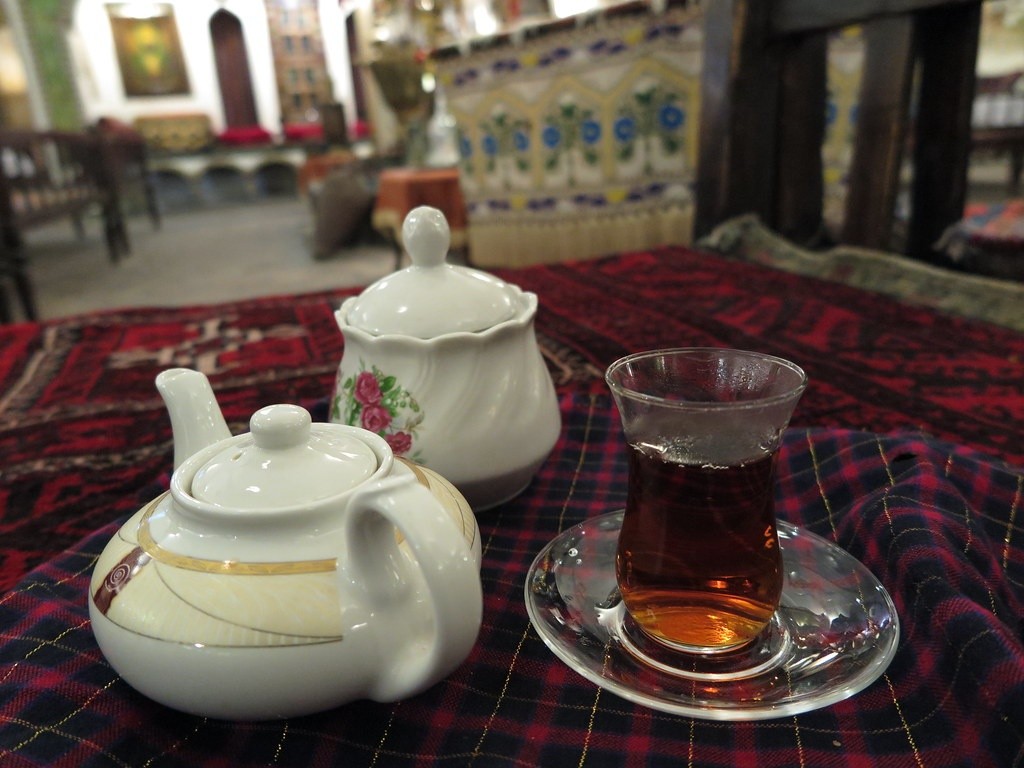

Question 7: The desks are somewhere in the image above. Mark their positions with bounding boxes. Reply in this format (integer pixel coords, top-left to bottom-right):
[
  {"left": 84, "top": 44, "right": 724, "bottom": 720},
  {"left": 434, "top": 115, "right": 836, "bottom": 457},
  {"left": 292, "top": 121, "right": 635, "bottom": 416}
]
[{"left": 0, "top": 214, "right": 1024, "bottom": 768}]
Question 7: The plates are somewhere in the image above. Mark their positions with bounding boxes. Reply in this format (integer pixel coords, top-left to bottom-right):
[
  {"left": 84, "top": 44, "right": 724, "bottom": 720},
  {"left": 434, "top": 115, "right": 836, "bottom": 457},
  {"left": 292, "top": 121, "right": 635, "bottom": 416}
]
[{"left": 523, "top": 508, "right": 900, "bottom": 723}]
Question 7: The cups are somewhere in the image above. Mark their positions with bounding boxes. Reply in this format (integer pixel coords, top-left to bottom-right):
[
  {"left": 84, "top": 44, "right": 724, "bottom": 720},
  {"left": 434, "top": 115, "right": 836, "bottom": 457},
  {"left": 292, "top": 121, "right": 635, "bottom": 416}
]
[
  {"left": 328, "top": 204, "right": 564, "bottom": 514},
  {"left": 605, "top": 344, "right": 808, "bottom": 657}
]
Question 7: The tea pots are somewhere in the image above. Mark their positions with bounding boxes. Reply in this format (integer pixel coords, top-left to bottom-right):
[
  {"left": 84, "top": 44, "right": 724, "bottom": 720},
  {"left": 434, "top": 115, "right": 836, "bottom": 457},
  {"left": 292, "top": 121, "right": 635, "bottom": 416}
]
[{"left": 88, "top": 368, "right": 486, "bottom": 726}]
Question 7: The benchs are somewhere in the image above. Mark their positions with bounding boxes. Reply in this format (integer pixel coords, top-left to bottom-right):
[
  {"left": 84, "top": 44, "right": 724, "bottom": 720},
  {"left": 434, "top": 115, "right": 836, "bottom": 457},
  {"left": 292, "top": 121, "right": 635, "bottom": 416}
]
[{"left": 0, "top": 127, "right": 130, "bottom": 322}]
